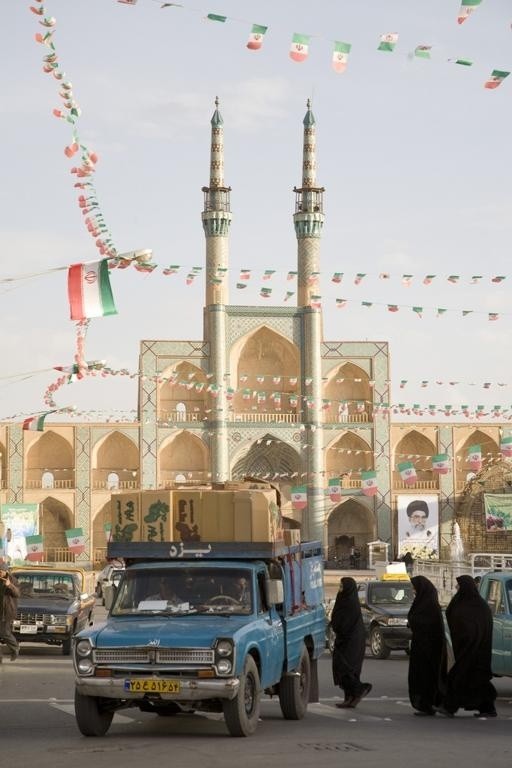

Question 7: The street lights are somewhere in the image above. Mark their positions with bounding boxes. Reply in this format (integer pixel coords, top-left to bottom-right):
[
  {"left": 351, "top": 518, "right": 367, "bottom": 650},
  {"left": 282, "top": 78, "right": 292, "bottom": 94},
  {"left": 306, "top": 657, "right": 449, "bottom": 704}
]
[
  {"left": 0, "top": 244, "right": 152, "bottom": 282},
  {"left": 1, "top": 404, "right": 77, "bottom": 430},
  {"left": 0, "top": 358, "right": 107, "bottom": 390}
]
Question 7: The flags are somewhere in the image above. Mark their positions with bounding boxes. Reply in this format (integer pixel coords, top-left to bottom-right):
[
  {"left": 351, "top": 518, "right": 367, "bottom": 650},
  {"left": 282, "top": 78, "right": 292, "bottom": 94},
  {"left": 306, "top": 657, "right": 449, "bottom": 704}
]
[
  {"left": 67, "top": 252, "right": 125, "bottom": 319},
  {"left": 20, "top": 410, "right": 56, "bottom": 432}
]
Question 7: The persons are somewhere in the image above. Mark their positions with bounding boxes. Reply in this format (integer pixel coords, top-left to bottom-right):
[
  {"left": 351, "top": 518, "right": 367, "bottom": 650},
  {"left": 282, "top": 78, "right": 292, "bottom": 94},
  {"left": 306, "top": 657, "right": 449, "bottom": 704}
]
[
  {"left": 147, "top": 575, "right": 185, "bottom": 608},
  {"left": 406, "top": 576, "right": 449, "bottom": 715},
  {"left": 218, "top": 574, "right": 251, "bottom": 608},
  {"left": 431, "top": 576, "right": 498, "bottom": 718},
  {"left": 398, "top": 501, "right": 437, "bottom": 550},
  {"left": 331, "top": 576, "right": 371, "bottom": 708},
  {"left": 0, "top": 562, "right": 21, "bottom": 660}
]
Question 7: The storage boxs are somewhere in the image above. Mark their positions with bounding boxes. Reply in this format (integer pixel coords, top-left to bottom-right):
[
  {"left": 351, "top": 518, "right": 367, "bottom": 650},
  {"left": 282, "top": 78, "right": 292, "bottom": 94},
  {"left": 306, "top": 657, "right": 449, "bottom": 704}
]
[{"left": 110, "top": 475, "right": 301, "bottom": 558}]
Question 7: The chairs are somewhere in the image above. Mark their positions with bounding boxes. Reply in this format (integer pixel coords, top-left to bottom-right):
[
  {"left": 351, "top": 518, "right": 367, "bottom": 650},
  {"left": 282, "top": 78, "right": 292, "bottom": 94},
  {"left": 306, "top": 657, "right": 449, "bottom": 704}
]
[
  {"left": 53, "top": 582, "right": 69, "bottom": 595},
  {"left": 20, "top": 581, "right": 34, "bottom": 596}
]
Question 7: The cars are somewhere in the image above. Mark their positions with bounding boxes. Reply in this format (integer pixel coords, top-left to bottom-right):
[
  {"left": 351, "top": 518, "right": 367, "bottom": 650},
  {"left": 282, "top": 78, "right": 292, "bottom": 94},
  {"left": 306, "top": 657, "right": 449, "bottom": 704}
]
[{"left": 94, "top": 563, "right": 128, "bottom": 598}]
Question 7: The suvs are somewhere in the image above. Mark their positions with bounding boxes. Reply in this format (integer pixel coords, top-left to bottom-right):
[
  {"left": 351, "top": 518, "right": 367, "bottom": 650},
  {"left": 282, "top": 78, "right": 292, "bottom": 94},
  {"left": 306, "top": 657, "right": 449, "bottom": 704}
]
[{"left": 321, "top": 577, "right": 419, "bottom": 657}]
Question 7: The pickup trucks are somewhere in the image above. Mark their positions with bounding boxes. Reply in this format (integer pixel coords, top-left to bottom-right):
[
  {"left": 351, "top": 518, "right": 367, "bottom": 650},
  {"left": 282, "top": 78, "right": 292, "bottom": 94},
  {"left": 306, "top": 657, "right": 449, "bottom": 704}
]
[{"left": 441, "top": 570, "right": 512, "bottom": 679}]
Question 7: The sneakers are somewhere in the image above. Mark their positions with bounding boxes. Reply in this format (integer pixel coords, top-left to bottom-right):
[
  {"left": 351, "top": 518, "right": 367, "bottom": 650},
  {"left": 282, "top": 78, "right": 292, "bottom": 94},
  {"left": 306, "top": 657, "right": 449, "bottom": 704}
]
[
  {"left": 10, "top": 641, "right": 20, "bottom": 662},
  {"left": 413, "top": 710, "right": 437, "bottom": 716},
  {"left": 431, "top": 704, "right": 455, "bottom": 719},
  {"left": 474, "top": 712, "right": 498, "bottom": 721},
  {"left": 335, "top": 683, "right": 372, "bottom": 709}
]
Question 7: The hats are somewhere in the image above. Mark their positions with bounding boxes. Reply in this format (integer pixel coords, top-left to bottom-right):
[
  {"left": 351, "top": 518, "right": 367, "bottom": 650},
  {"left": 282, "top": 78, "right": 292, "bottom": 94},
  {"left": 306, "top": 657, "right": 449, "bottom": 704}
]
[{"left": 406, "top": 500, "right": 429, "bottom": 518}]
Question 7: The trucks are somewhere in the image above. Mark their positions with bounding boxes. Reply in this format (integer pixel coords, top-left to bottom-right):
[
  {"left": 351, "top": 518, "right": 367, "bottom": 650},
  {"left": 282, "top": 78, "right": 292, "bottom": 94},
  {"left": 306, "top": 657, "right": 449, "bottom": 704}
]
[
  {"left": 71, "top": 534, "right": 337, "bottom": 736},
  {"left": 5, "top": 565, "right": 96, "bottom": 657}
]
